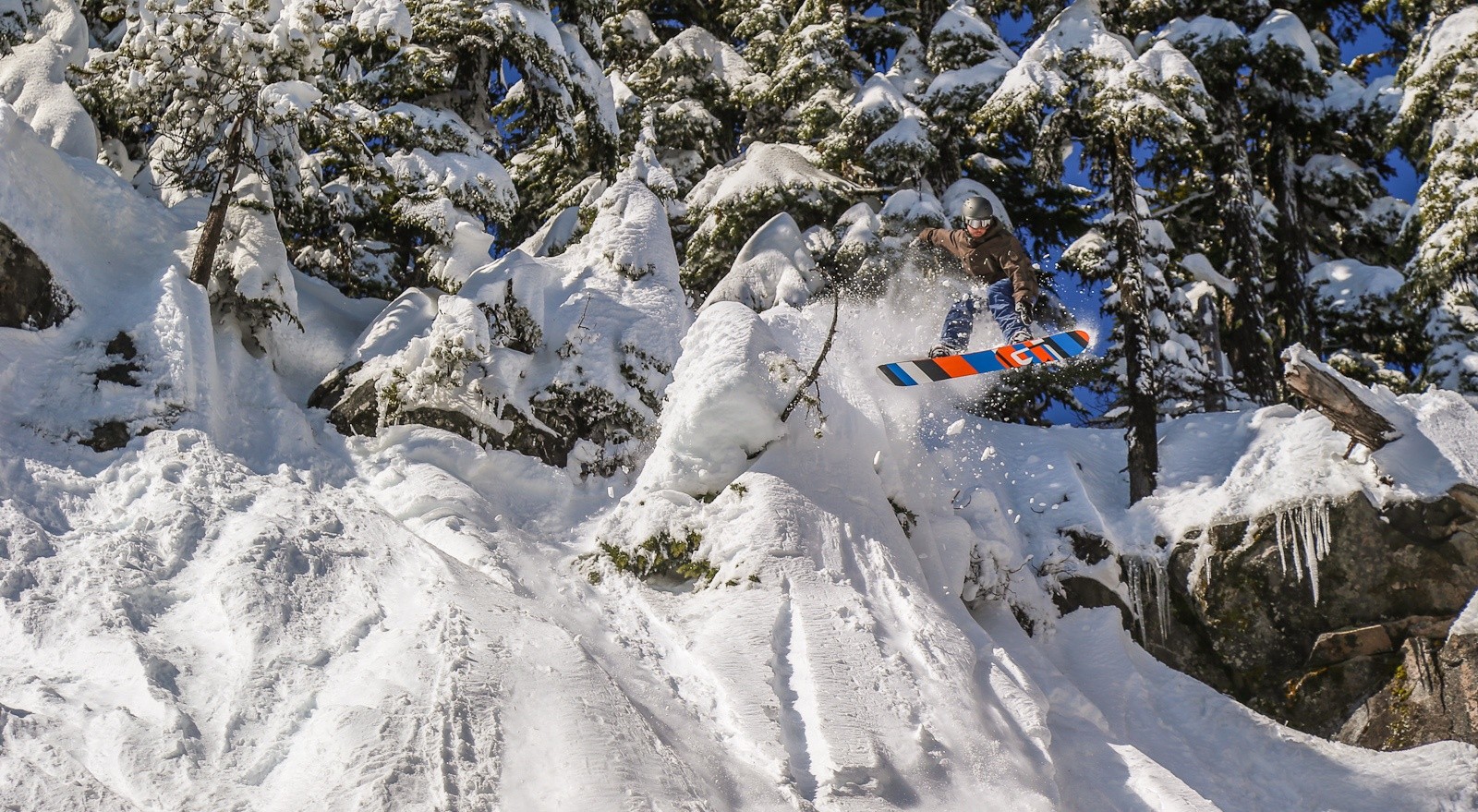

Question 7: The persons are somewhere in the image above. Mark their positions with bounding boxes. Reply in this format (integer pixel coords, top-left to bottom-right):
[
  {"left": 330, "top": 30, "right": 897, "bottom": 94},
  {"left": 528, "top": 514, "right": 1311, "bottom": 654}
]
[{"left": 908, "top": 197, "right": 1036, "bottom": 358}]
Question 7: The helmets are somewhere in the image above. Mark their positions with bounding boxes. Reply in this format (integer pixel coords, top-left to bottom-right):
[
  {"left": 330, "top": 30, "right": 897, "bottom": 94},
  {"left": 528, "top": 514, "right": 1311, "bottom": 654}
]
[{"left": 962, "top": 196, "right": 993, "bottom": 219}]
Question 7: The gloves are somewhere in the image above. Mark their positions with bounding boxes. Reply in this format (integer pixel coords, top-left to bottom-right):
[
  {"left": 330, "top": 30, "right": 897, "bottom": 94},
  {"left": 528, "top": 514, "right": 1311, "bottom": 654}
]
[
  {"left": 1014, "top": 301, "right": 1034, "bottom": 326},
  {"left": 909, "top": 236, "right": 924, "bottom": 249}
]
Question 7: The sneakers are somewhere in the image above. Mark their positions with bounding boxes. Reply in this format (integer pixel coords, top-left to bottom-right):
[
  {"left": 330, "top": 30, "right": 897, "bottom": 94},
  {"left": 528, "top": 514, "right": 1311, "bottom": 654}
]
[
  {"left": 1011, "top": 333, "right": 1034, "bottom": 344},
  {"left": 928, "top": 344, "right": 957, "bottom": 358}
]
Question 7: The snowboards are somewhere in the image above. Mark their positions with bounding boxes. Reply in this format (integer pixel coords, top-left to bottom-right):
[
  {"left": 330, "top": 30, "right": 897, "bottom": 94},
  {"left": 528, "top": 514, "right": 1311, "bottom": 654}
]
[{"left": 875, "top": 330, "right": 1089, "bottom": 386}]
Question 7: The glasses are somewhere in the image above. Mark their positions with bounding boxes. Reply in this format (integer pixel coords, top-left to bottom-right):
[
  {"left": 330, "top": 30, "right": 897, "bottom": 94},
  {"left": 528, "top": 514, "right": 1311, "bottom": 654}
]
[{"left": 965, "top": 219, "right": 992, "bottom": 229}]
